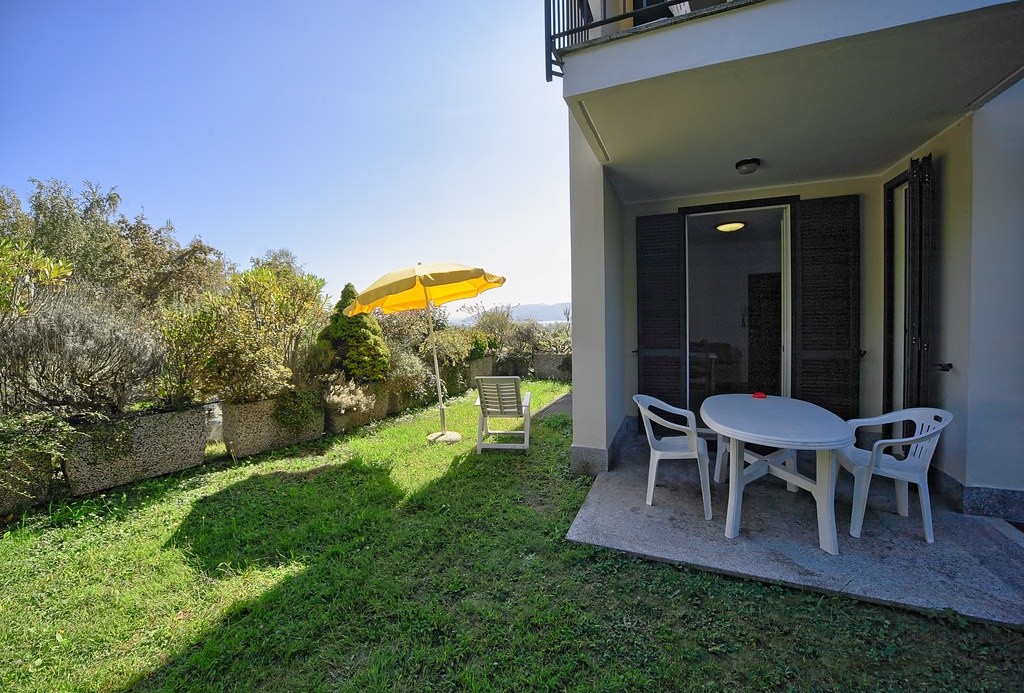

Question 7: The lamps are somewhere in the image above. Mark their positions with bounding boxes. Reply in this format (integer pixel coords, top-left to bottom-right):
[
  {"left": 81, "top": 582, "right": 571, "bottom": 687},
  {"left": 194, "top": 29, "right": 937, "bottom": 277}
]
[
  {"left": 734, "top": 157, "right": 762, "bottom": 175},
  {"left": 714, "top": 221, "right": 745, "bottom": 233}
]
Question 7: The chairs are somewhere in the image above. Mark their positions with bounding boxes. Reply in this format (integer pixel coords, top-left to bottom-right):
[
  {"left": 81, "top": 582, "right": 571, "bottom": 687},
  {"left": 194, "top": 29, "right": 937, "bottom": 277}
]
[
  {"left": 473, "top": 375, "right": 532, "bottom": 455},
  {"left": 633, "top": 394, "right": 712, "bottom": 522},
  {"left": 835, "top": 407, "right": 954, "bottom": 543}
]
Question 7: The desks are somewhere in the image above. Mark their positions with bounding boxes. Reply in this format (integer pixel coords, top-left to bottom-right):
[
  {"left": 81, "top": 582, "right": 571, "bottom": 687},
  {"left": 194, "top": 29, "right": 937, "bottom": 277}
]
[{"left": 700, "top": 393, "right": 856, "bottom": 555}]
